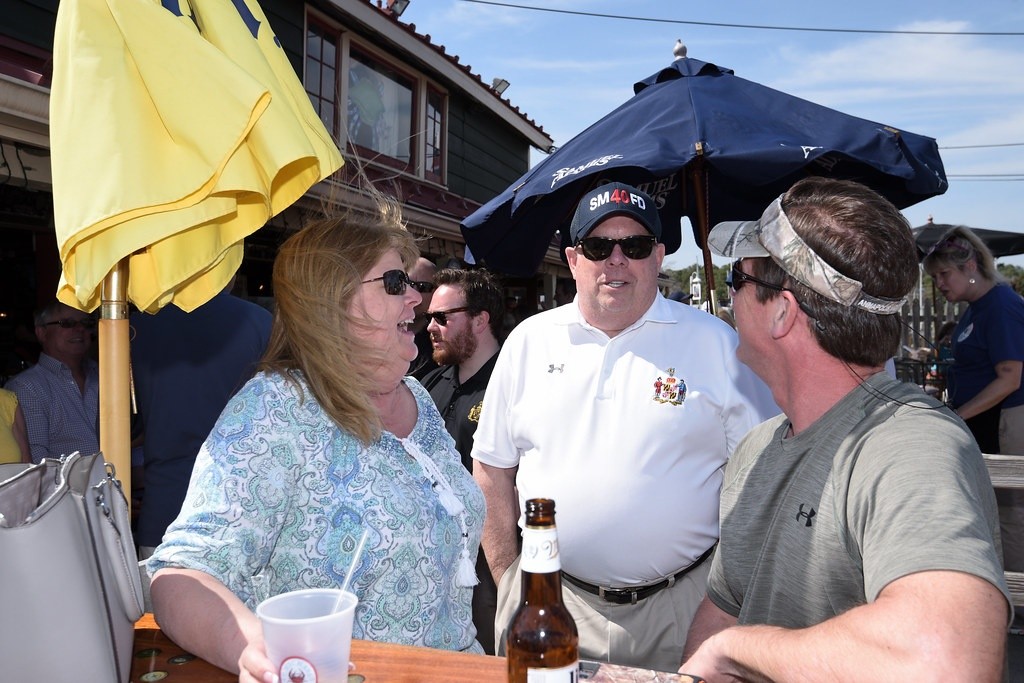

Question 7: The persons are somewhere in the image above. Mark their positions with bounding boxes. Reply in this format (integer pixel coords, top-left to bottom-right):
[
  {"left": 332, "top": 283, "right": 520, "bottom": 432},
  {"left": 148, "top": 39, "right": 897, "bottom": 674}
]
[
  {"left": 923, "top": 322, "right": 958, "bottom": 384},
  {"left": 666, "top": 290, "right": 692, "bottom": 309},
  {"left": 404, "top": 257, "right": 440, "bottom": 384},
  {"left": 922, "top": 224, "right": 1024, "bottom": 455},
  {"left": 551, "top": 276, "right": 576, "bottom": 307},
  {"left": 131, "top": 272, "right": 275, "bottom": 562},
  {"left": 128, "top": 219, "right": 488, "bottom": 683},
  {"left": 470, "top": 182, "right": 786, "bottom": 666},
  {"left": 0, "top": 387, "right": 33, "bottom": 464},
  {"left": 680, "top": 179, "right": 1014, "bottom": 683},
  {"left": 497, "top": 292, "right": 543, "bottom": 341},
  {"left": 5, "top": 301, "right": 99, "bottom": 463},
  {"left": 417, "top": 268, "right": 513, "bottom": 476}
]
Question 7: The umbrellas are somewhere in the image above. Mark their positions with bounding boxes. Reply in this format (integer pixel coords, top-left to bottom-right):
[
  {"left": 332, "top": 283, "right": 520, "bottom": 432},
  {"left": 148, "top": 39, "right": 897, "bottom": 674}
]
[
  {"left": 49, "top": 0, "right": 344, "bottom": 549},
  {"left": 910, "top": 223, "right": 1024, "bottom": 262},
  {"left": 459, "top": 59, "right": 949, "bottom": 324}
]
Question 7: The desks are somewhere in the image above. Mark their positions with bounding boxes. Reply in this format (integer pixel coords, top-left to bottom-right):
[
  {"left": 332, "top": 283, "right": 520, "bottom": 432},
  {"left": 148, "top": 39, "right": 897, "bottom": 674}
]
[{"left": 126, "top": 614, "right": 706, "bottom": 683}]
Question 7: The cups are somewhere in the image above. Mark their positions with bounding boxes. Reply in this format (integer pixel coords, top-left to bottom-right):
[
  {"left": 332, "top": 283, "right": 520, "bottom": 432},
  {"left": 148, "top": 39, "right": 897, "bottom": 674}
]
[{"left": 255, "top": 588, "right": 359, "bottom": 683}]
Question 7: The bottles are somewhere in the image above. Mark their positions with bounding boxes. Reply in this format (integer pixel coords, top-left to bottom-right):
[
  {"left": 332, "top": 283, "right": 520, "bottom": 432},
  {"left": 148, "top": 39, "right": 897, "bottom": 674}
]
[{"left": 505, "top": 498, "right": 581, "bottom": 683}]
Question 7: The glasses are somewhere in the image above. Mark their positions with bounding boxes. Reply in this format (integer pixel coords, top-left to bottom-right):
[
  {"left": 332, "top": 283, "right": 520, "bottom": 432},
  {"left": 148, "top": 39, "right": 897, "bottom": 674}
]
[
  {"left": 731, "top": 261, "right": 792, "bottom": 291},
  {"left": 574, "top": 235, "right": 657, "bottom": 261},
  {"left": 359, "top": 270, "right": 411, "bottom": 296},
  {"left": 411, "top": 280, "right": 437, "bottom": 295},
  {"left": 423, "top": 306, "right": 471, "bottom": 328},
  {"left": 40, "top": 317, "right": 97, "bottom": 330}
]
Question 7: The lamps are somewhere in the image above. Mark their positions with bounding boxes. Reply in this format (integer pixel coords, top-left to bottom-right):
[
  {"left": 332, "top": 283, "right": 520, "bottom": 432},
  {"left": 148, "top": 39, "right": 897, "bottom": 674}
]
[
  {"left": 493, "top": 78, "right": 510, "bottom": 94},
  {"left": 387, "top": 0, "right": 410, "bottom": 16}
]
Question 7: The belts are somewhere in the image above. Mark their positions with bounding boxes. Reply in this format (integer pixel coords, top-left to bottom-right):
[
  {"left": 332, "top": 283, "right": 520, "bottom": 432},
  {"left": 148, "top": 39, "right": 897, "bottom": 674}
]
[{"left": 559, "top": 540, "right": 722, "bottom": 605}]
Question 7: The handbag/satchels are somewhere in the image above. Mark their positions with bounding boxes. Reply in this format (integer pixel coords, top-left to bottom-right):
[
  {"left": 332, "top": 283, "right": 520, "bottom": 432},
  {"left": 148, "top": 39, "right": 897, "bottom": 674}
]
[{"left": 0, "top": 450, "right": 144, "bottom": 683}]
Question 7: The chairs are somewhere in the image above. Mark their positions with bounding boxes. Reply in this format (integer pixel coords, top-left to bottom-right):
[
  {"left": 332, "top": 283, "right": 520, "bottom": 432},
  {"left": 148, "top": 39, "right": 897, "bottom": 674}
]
[{"left": 894, "top": 358, "right": 940, "bottom": 395}]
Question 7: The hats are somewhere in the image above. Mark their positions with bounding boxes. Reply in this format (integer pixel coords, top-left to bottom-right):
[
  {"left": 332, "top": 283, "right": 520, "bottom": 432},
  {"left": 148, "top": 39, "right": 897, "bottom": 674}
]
[
  {"left": 570, "top": 182, "right": 661, "bottom": 245},
  {"left": 707, "top": 191, "right": 906, "bottom": 315},
  {"left": 666, "top": 289, "right": 692, "bottom": 302}
]
